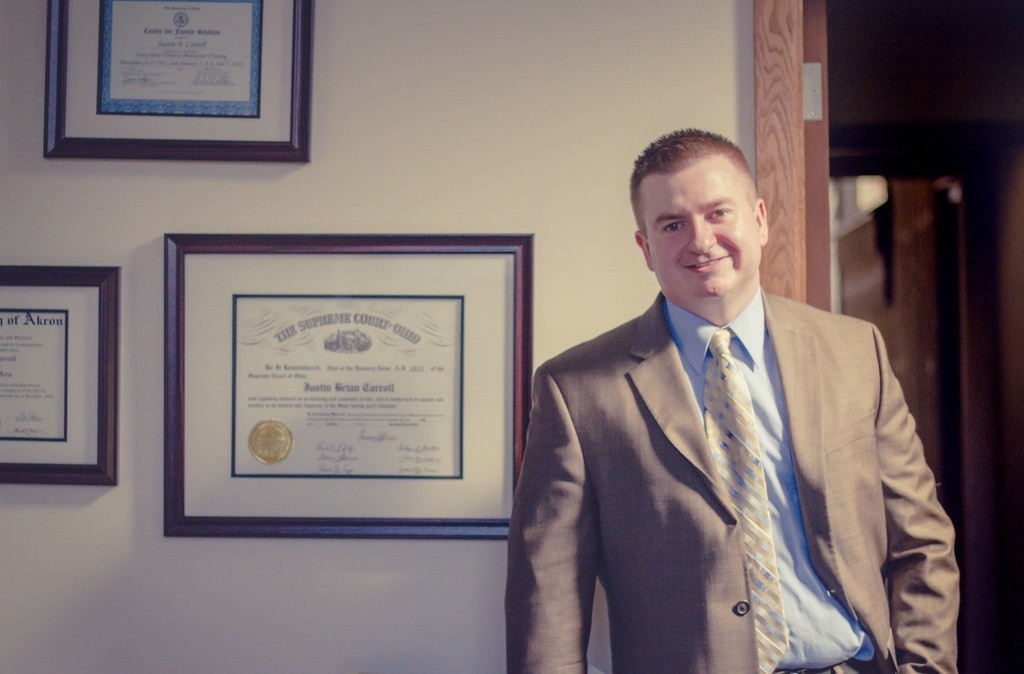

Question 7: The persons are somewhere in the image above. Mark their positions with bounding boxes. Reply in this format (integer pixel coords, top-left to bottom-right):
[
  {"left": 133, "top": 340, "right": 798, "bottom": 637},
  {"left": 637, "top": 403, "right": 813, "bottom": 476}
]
[{"left": 505, "top": 128, "right": 961, "bottom": 674}]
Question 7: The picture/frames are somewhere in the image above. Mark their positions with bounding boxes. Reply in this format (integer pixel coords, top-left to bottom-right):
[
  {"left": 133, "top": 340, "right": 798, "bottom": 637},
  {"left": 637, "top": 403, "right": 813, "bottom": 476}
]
[
  {"left": 0, "top": 263, "right": 118, "bottom": 484},
  {"left": 166, "top": 228, "right": 523, "bottom": 538},
  {"left": 42, "top": 0, "right": 311, "bottom": 162}
]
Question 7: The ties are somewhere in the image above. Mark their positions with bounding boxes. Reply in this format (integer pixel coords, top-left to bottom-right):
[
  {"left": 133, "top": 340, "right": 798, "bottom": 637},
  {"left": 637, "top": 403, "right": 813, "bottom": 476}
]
[{"left": 703, "top": 330, "right": 790, "bottom": 674}]
